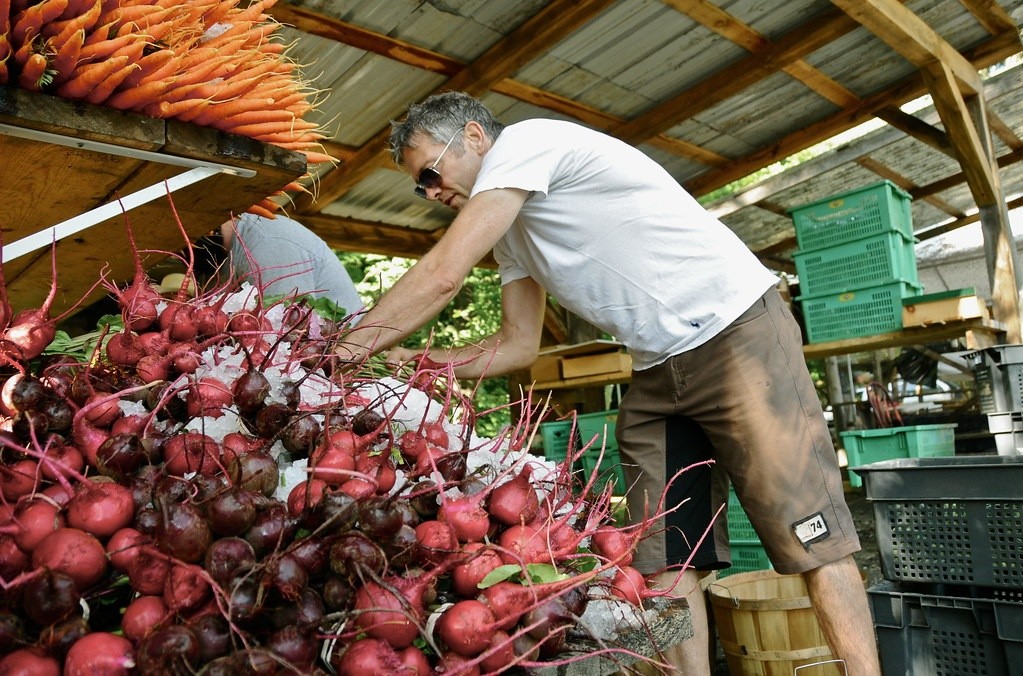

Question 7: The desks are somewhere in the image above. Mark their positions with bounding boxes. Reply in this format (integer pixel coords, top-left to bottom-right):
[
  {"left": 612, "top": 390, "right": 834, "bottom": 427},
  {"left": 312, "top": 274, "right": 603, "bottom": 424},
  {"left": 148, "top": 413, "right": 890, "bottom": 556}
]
[
  {"left": 0, "top": 83, "right": 306, "bottom": 323},
  {"left": 524, "top": 319, "right": 1012, "bottom": 414}
]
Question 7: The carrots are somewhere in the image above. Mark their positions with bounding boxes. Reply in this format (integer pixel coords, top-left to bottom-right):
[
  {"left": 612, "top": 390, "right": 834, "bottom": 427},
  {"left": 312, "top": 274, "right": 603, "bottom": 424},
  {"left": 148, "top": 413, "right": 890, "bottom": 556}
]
[{"left": 0, "top": 0, "right": 727, "bottom": 220}]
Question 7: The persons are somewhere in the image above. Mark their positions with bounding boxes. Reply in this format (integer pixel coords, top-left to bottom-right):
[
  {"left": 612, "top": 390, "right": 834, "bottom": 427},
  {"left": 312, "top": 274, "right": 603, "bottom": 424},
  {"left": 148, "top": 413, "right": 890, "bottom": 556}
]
[
  {"left": 191, "top": 210, "right": 363, "bottom": 337},
  {"left": 330, "top": 93, "right": 884, "bottom": 676}
]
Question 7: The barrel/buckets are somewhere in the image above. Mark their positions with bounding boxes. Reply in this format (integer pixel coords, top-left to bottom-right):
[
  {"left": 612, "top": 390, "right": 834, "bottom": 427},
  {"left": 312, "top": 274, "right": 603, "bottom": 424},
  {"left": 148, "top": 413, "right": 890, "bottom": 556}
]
[{"left": 707, "top": 566, "right": 871, "bottom": 676}]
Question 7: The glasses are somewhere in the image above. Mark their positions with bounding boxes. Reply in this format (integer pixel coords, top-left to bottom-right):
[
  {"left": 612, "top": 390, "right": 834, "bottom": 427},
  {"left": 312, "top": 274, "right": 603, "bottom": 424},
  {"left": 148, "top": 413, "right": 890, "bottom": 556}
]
[{"left": 414, "top": 127, "right": 464, "bottom": 201}]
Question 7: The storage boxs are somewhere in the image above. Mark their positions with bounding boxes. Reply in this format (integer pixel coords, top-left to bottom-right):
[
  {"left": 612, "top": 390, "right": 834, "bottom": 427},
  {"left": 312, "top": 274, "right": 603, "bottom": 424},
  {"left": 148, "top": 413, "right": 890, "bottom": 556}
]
[
  {"left": 529, "top": 343, "right": 632, "bottom": 381},
  {"left": 524, "top": 407, "right": 625, "bottom": 498},
  {"left": 786, "top": 179, "right": 982, "bottom": 345},
  {"left": 842, "top": 344, "right": 1023, "bottom": 676},
  {"left": 718, "top": 477, "right": 773, "bottom": 577}
]
